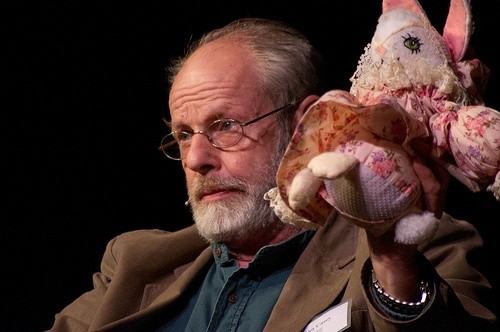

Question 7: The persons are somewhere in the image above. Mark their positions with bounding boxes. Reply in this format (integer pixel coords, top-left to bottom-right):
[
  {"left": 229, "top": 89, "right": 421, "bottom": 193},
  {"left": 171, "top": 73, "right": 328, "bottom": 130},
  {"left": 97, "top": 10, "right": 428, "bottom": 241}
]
[{"left": 48, "top": 19, "right": 500, "bottom": 332}]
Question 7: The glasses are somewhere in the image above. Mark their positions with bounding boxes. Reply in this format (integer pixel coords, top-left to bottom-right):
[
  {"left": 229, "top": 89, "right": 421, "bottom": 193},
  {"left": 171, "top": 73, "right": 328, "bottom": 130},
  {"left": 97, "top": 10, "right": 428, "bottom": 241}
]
[{"left": 160, "top": 100, "right": 303, "bottom": 160}]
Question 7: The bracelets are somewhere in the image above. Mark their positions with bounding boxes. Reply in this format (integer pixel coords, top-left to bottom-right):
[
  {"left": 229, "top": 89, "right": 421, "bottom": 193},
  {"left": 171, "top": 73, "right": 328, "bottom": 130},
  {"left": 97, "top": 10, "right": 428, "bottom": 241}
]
[{"left": 368, "top": 267, "right": 433, "bottom": 315}]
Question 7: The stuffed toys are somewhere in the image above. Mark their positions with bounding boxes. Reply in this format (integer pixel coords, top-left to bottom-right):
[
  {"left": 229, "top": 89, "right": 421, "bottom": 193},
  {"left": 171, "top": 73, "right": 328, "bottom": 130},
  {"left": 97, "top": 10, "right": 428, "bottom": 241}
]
[{"left": 263, "top": 0, "right": 500, "bottom": 247}]
